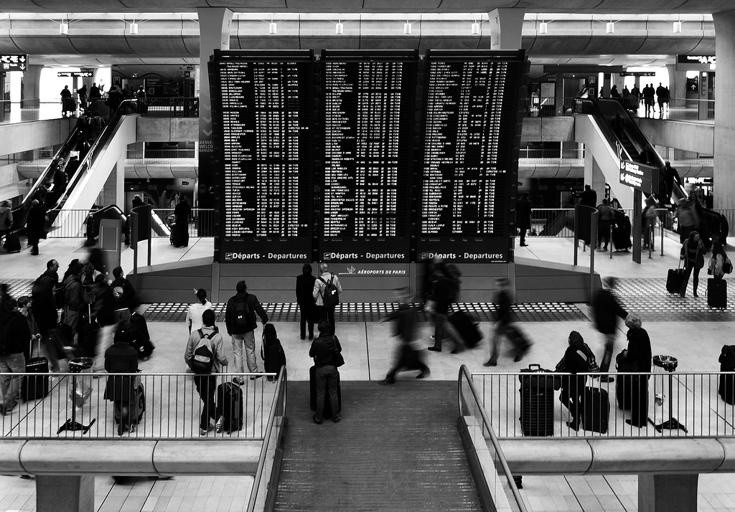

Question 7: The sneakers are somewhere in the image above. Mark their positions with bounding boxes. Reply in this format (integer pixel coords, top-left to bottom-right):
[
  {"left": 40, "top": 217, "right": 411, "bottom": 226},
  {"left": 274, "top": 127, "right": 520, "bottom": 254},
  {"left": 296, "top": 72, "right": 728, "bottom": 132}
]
[
  {"left": 201, "top": 425, "right": 213, "bottom": 435},
  {"left": 215, "top": 415, "right": 225, "bottom": 432},
  {"left": 0, "top": 399, "right": 17, "bottom": 416}
]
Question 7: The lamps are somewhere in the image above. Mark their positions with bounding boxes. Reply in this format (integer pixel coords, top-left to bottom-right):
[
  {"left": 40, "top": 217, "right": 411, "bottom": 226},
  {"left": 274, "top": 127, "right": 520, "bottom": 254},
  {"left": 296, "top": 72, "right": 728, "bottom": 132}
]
[{"left": 49, "top": 12, "right": 685, "bottom": 37}]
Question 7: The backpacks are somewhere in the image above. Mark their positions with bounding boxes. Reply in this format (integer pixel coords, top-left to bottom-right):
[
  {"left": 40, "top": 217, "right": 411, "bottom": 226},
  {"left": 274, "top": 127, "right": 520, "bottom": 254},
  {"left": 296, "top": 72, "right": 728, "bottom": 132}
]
[
  {"left": 318, "top": 272, "right": 339, "bottom": 308},
  {"left": 191, "top": 329, "right": 219, "bottom": 373},
  {"left": 722, "top": 256, "right": 733, "bottom": 275}
]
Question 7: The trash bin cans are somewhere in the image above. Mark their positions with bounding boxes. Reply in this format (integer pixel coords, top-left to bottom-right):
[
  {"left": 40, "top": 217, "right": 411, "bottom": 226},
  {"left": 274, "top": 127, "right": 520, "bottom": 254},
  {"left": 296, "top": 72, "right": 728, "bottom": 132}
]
[
  {"left": 651, "top": 352, "right": 678, "bottom": 409},
  {"left": 68, "top": 358, "right": 93, "bottom": 402}
]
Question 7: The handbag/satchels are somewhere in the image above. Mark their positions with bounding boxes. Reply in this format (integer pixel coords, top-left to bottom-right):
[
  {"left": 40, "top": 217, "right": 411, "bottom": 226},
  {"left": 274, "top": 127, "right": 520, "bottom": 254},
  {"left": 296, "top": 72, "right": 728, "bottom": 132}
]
[
  {"left": 616, "top": 351, "right": 629, "bottom": 372},
  {"left": 263, "top": 324, "right": 277, "bottom": 345},
  {"left": 332, "top": 347, "right": 344, "bottom": 367},
  {"left": 718, "top": 345, "right": 734, "bottom": 366},
  {"left": 588, "top": 356, "right": 601, "bottom": 378},
  {"left": 128, "top": 368, "right": 141, "bottom": 390},
  {"left": 519, "top": 369, "right": 553, "bottom": 395}
]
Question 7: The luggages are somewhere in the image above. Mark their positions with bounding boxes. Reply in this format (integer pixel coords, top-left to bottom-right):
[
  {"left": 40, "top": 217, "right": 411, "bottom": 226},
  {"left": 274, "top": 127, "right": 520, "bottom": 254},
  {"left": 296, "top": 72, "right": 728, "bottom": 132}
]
[
  {"left": 615, "top": 369, "right": 631, "bottom": 411},
  {"left": 719, "top": 365, "right": 734, "bottom": 406},
  {"left": 20, "top": 335, "right": 48, "bottom": 403},
  {"left": 707, "top": 264, "right": 726, "bottom": 307},
  {"left": 260, "top": 321, "right": 286, "bottom": 379},
  {"left": 130, "top": 382, "right": 145, "bottom": 425},
  {"left": 666, "top": 258, "right": 686, "bottom": 298},
  {"left": 449, "top": 303, "right": 482, "bottom": 348},
  {"left": 519, "top": 364, "right": 553, "bottom": 437},
  {"left": 217, "top": 364, "right": 243, "bottom": 434},
  {"left": 310, "top": 365, "right": 341, "bottom": 413},
  {"left": 581, "top": 372, "right": 610, "bottom": 434}
]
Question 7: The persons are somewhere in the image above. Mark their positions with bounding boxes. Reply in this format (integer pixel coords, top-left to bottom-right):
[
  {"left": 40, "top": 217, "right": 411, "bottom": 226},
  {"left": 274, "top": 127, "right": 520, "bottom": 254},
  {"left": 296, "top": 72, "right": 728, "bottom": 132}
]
[
  {"left": 383, "top": 251, "right": 529, "bottom": 384},
  {"left": 611, "top": 84, "right": 671, "bottom": 118},
  {"left": 0, "top": 248, "right": 153, "bottom": 435},
  {"left": 185, "top": 288, "right": 212, "bottom": 336},
  {"left": 185, "top": 309, "right": 228, "bottom": 435},
  {"left": 61, "top": 84, "right": 148, "bottom": 159},
  {"left": 308, "top": 320, "right": 344, "bottom": 424},
  {"left": 517, "top": 161, "right": 728, "bottom": 296},
  {"left": 132, "top": 194, "right": 142, "bottom": 207},
  {"left": 170, "top": 192, "right": 191, "bottom": 247},
  {"left": 1, "top": 158, "right": 69, "bottom": 255},
  {"left": 554, "top": 279, "right": 653, "bottom": 435},
  {"left": 225, "top": 280, "right": 268, "bottom": 385},
  {"left": 296, "top": 262, "right": 341, "bottom": 340}
]
